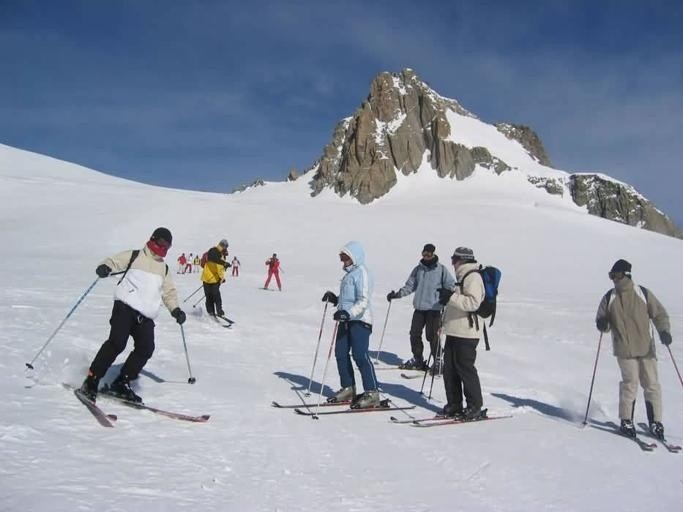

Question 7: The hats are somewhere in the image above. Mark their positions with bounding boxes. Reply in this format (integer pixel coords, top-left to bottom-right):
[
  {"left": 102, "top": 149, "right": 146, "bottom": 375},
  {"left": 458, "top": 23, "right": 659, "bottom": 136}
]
[
  {"left": 611, "top": 260, "right": 631, "bottom": 279},
  {"left": 423, "top": 244, "right": 435, "bottom": 255},
  {"left": 150, "top": 228, "right": 172, "bottom": 245},
  {"left": 220, "top": 239, "right": 228, "bottom": 248},
  {"left": 452, "top": 246, "right": 474, "bottom": 261}
]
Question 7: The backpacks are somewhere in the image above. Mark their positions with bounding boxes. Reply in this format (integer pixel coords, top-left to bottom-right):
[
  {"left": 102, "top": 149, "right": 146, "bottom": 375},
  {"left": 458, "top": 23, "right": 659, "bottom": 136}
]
[{"left": 459, "top": 264, "right": 503, "bottom": 319}]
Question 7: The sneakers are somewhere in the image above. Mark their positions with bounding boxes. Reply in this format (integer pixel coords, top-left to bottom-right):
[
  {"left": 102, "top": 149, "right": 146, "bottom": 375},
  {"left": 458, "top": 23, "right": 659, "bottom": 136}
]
[
  {"left": 327, "top": 386, "right": 356, "bottom": 402},
  {"left": 403, "top": 358, "right": 423, "bottom": 367},
  {"left": 82, "top": 375, "right": 98, "bottom": 402},
  {"left": 458, "top": 405, "right": 481, "bottom": 419},
  {"left": 110, "top": 375, "right": 141, "bottom": 403},
  {"left": 620, "top": 421, "right": 636, "bottom": 437},
  {"left": 650, "top": 423, "right": 665, "bottom": 440},
  {"left": 443, "top": 403, "right": 463, "bottom": 414},
  {"left": 352, "top": 390, "right": 380, "bottom": 408}
]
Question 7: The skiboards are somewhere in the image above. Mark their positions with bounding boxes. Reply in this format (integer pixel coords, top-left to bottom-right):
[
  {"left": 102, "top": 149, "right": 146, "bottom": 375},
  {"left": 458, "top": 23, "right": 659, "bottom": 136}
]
[
  {"left": 258, "top": 288, "right": 282, "bottom": 291},
  {"left": 63, "top": 383, "right": 210, "bottom": 428},
  {"left": 353, "top": 366, "right": 441, "bottom": 379},
  {"left": 209, "top": 315, "right": 234, "bottom": 327},
  {"left": 391, "top": 411, "right": 514, "bottom": 426},
  {"left": 606, "top": 421, "right": 682, "bottom": 451},
  {"left": 271, "top": 398, "right": 415, "bottom": 415}
]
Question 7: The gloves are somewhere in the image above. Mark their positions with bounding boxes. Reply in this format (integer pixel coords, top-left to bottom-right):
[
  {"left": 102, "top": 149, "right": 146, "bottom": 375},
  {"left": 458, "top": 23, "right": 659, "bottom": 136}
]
[
  {"left": 95, "top": 265, "right": 111, "bottom": 277},
  {"left": 386, "top": 291, "right": 398, "bottom": 300},
  {"left": 171, "top": 308, "right": 186, "bottom": 324},
  {"left": 660, "top": 332, "right": 672, "bottom": 344},
  {"left": 437, "top": 288, "right": 448, "bottom": 298},
  {"left": 334, "top": 310, "right": 349, "bottom": 321},
  {"left": 321, "top": 292, "right": 337, "bottom": 305},
  {"left": 597, "top": 320, "right": 610, "bottom": 331}
]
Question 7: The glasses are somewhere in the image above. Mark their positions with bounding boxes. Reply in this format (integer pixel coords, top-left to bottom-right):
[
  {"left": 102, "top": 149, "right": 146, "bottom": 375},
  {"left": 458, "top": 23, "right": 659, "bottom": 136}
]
[{"left": 609, "top": 272, "right": 625, "bottom": 279}]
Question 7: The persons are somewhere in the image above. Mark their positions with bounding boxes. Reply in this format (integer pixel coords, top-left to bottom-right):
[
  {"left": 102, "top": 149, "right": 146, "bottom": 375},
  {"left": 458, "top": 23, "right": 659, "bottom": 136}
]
[
  {"left": 230, "top": 257, "right": 240, "bottom": 276},
  {"left": 80, "top": 228, "right": 186, "bottom": 402},
  {"left": 202, "top": 240, "right": 228, "bottom": 316},
  {"left": 177, "top": 253, "right": 201, "bottom": 274},
  {"left": 265, "top": 254, "right": 282, "bottom": 291},
  {"left": 437, "top": 248, "right": 486, "bottom": 420},
  {"left": 387, "top": 245, "right": 455, "bottom": 374},
  {"left": 596, "top": 260, "right": 672, "bottom": 438},
  {"left": 322, "top": 243, "right": 380, "bottom": 408}
]
